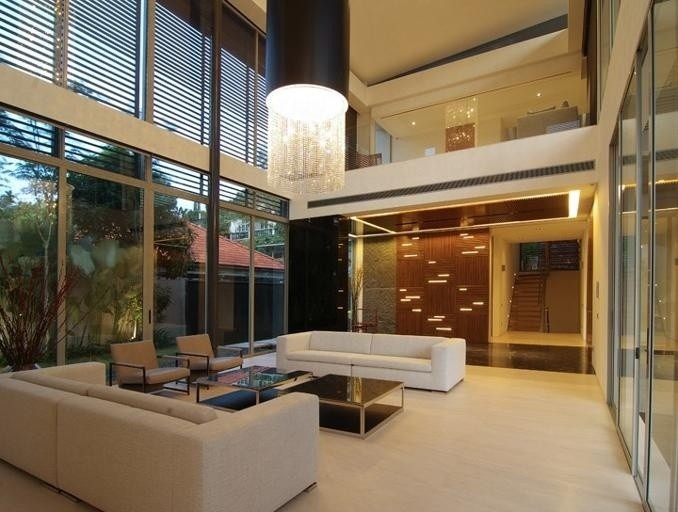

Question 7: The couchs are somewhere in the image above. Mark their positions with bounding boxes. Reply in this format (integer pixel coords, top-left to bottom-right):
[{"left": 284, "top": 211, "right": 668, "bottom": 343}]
[
  {"left": 0, "top": 361, "right": 319, "bottom": 512},
  {"left": 276, "top": 330, "right": 466, "bottom": 393}
]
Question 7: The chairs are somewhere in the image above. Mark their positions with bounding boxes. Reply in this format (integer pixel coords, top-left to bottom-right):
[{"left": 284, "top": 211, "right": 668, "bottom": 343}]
[
  {"left": 352, "top": 307, "right": 379, "bottom": 333},
  {"left": 507, "top": 100, "right": 580, "bottom": 140},
  {"left": 175, "top": 333, "right": 244, "bottom": 389},
  {"left": 108, "top": 340, "right": 191, "bottom": 395}
]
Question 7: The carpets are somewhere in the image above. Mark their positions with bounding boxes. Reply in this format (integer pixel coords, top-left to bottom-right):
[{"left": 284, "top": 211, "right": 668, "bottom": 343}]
[{"left": 154, "top": 365, "right": 319, "bottom": 413}]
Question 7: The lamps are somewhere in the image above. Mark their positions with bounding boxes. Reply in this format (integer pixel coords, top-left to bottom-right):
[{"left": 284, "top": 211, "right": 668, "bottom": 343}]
[{"left": 263, "top": 0, "right": 349, "bottom": 196}]
